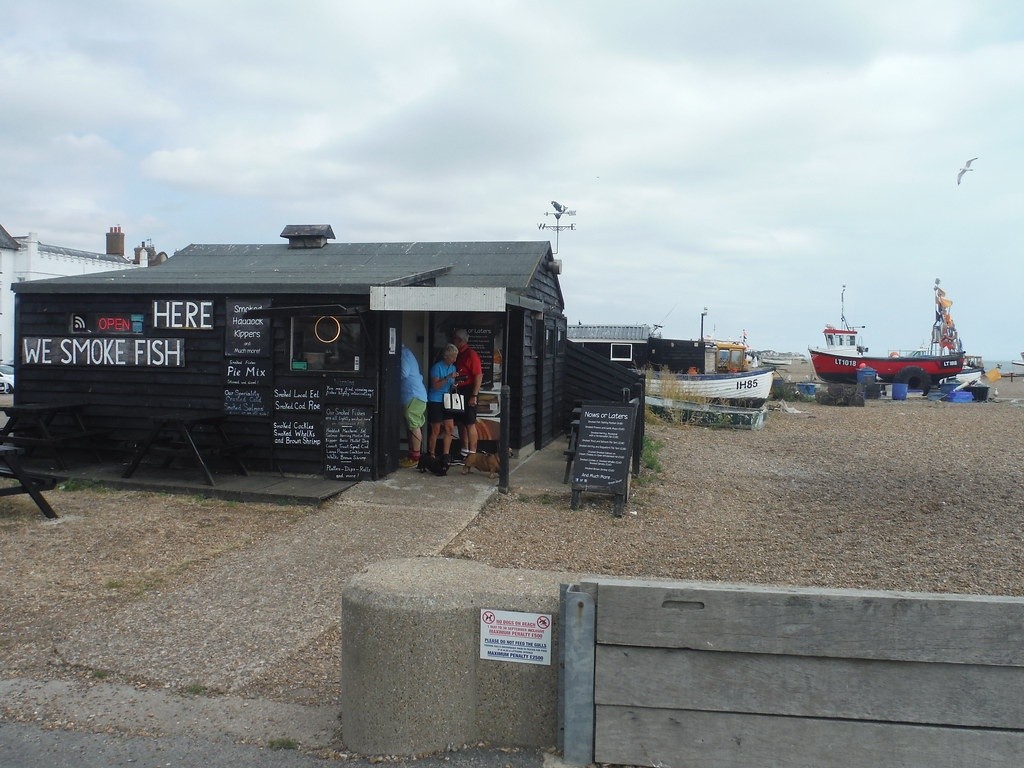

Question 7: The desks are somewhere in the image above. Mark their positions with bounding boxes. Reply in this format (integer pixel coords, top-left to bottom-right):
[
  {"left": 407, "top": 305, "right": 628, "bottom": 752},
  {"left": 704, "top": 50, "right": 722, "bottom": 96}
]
[
  {"left": 0, "top": 444, "right": 59, "bottom": 518},
  {"left": 122, "top": 414, "right": 249, "bottom": 487},
  {"left": 0, "top": 403, "right": 102, "bottom": 472}
]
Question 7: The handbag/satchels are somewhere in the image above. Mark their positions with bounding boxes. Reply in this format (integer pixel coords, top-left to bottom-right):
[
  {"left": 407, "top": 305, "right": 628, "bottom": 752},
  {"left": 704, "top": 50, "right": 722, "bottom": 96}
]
[{"left": 444, "top": 386, "right": 465, "bottom": 413}]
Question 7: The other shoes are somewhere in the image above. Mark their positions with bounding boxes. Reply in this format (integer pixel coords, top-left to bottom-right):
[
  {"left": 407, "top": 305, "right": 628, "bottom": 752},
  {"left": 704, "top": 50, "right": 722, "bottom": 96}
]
[
  {"left": 442, "top": 453, "right": 449, "bottom": 467},
  {"left": 399, "top": 455, "right": 420, "bottom": 468},
  {"left": 449, "top": 452, "right": 474, "bottom": 466}
]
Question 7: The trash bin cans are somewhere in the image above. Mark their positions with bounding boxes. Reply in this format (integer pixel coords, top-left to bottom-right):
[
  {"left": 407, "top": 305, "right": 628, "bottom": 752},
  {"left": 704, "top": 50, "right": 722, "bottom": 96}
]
[{"left": 856, "top": 367, "right": 876, "bottom": 385}]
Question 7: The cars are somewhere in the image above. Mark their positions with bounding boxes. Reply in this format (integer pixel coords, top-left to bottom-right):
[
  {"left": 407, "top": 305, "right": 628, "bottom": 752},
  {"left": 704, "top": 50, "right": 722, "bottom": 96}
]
[{"left": 0, "top": 365, "right": 14, "bottom": 395}]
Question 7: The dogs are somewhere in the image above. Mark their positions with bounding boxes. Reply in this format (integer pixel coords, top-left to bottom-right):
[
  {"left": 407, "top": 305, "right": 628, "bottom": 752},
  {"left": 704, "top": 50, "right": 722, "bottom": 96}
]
[
  {"left": 416, "top": 453, "right": 451, "bottom": 476},
  {"left": 462, "top": 447, "right": 513, "bottom": 479}
]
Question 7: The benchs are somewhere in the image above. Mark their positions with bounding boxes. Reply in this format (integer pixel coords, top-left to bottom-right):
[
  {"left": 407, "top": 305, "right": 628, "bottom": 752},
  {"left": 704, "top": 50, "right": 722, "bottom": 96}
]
[{"left": 0, "top": 425, "right": 254, "bottom": 518}]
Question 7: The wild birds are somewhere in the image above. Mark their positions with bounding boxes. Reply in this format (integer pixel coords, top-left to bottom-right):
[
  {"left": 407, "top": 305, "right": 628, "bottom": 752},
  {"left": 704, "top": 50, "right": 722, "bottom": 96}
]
[{"left": 957, "top": 157, "right": 978, "bottom": 185}]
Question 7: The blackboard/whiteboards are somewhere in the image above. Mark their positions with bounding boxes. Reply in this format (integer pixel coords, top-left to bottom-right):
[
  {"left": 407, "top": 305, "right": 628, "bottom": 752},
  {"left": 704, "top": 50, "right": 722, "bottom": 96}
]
[
  {"left": 321, "top": 379, "right": 374, "bottom": 480},
  {"left": 273, "top": 384, "right": 321, "bottom": 448},
  {"left": 225, "top": 297, "right": 273, "bottom": 356},
  {"left": 571, "top": 404, "right": 638, "bottom": 495},
  {"left": 223, "top": 358, "right": 273, "bottom": 420}
]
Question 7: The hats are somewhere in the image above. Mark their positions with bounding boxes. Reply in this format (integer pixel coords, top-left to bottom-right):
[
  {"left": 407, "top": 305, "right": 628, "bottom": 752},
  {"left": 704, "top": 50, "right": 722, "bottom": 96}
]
[{"left": 455, "top": 329, "right": 469, "bottom": 342}]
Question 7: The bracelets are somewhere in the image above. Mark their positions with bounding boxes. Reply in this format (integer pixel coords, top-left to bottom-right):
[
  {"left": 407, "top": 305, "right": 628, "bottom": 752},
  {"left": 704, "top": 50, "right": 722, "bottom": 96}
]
[
  {"left": 472, "top": 395, "right": 477, "bottom": 397},
  {"left": 445, "top": 376, "right": 448, "bottom": 381}
]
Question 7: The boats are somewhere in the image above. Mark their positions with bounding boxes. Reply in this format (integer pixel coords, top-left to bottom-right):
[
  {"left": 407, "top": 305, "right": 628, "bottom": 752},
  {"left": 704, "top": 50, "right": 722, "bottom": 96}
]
[
  {"left": 808, "top": 284, "right": 985, "bottom": 390},
  {"left": 627, "top": 364, "right": 776, "bottom": 408},
  {"left": 644, "top": 395, "right": 770, "bottom": 431},
  {"left": 762, "top": 358, "right": 792, "bottom": 366}
]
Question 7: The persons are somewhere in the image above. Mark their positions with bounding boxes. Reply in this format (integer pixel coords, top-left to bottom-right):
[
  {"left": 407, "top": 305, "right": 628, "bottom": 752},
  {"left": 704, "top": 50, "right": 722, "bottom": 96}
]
[
  {"left": 429, "top": 344, "right": 458, "bottom": 459},
  {"left": 449, "top": 329, "right": 483, "bottom": 466},
  {"left": 400, "top": 345, "right": 428, "bottom": 467}
]
